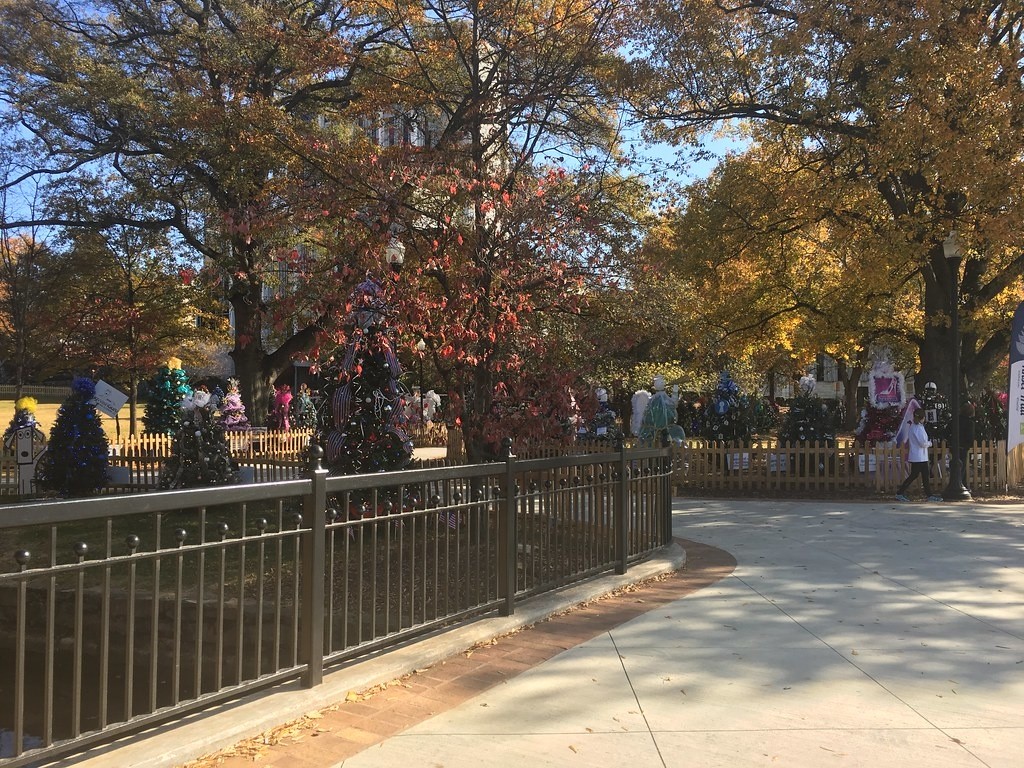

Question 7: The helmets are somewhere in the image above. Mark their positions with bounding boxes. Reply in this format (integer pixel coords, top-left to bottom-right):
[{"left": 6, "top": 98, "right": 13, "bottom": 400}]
[
  {"left": 594, "top": 388, "right": 606, "bottom": 399},
  {"left": 924, "top": 382, "right": 936, "bottom": 395}
]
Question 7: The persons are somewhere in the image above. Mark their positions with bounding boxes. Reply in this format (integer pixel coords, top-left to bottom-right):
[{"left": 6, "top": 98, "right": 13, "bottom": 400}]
[{"left": 895, "top": 408, "right": 943, "bottom": 501}]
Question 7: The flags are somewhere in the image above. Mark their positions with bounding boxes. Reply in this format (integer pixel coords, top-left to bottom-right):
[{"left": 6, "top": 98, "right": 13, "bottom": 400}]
[
  {"left": 440, "top": 510, "right": 463, "bottom": 529},
  {"left": 1006, "top": 299, "right": 1024, "bottom": 452},
  {"left": 350, "top": 526, "right": 355, "bottom": 542},
  {"left": 396, "top": 519, "right": 405, "bottom": 529}
]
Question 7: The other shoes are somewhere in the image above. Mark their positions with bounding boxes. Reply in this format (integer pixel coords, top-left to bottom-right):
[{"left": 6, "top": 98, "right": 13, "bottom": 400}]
[
  {"left": 895, "top": 494, "right": 910, "bottom": 502},
  {"left": 926, "top": 496, "right": 944, "bottom": 502}
]
